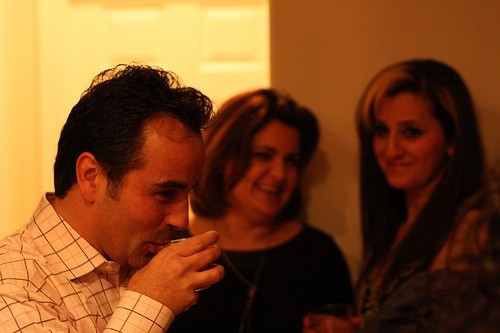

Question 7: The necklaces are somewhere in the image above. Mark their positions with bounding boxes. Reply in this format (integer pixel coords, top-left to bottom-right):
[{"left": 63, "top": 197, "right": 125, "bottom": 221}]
[{"left": 212, "top": 232, "right": 274, "bottom": 333}]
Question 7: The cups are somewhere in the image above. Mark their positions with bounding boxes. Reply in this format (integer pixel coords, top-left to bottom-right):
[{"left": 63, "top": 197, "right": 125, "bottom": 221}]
[{"left": 156, "top": 239, "right": 211, "bottom": 292}]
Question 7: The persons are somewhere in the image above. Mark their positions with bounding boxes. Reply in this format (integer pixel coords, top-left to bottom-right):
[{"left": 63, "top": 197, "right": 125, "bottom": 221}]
[
  {"left": 1, "top": 56, "right": 225, "bottom": 333},
  {"left": 301, "top": 58, "right": 499, "bottom": 333},
  {"left": 166, "top": 87, "right": 356, "bottom": 333}
]
